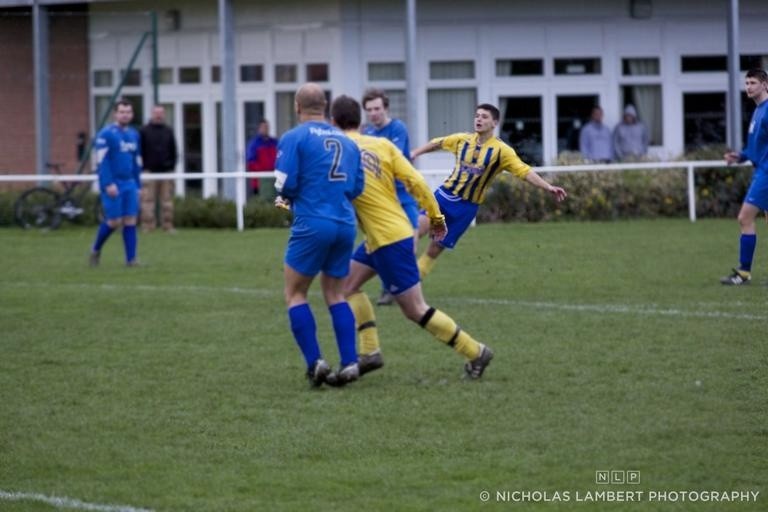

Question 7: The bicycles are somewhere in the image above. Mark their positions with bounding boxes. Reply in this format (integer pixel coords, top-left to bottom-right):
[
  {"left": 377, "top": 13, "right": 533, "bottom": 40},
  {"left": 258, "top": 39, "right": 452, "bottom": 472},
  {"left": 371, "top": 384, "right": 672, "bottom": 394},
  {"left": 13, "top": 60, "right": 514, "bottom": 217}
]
[{"left": 12, "top": 161, "right": 146, "bottom": 231}]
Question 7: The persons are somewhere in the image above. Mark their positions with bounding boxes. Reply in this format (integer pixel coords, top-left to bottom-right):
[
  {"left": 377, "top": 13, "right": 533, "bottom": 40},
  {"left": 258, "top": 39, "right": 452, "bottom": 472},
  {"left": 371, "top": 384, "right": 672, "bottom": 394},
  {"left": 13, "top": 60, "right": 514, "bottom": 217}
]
[
  {"left": 379, "top": 104, "right": 567, "bottom": 304},
  {"left": 578, "top": 105, "right": 615, "bottom": 163},
  {"left": 245, "top": 119, "right": 279, "bottom": 195},
  {"left": 359, "top": 90, "right": 419, "bottom": 256},
  {"left": 274, "top": 81, "right": 364, "bottom": 387},
  {"left": 719, "top": 68, "right": 768, "bottom": 286},
  {"left": 274, "top": 94, "right": 494, "bottom": 380},
  {"left": 611, "top": 104, "right": 650, "bottom": 163},
  {"left": 88, "top": 100, "right": 148, "bottom": 268},
  {"left": 139, "top": 106, "right": 176, "bottom": 234}
]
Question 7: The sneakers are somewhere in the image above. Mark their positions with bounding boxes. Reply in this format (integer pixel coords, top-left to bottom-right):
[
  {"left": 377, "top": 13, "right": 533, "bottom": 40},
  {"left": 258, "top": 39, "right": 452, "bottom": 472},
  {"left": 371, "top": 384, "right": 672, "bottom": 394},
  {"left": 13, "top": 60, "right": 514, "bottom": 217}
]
[
  {"left": 462, "top": 345, "right": 494, "bottom": 379},
  {"left": 377, "top": 290, "right": 393, "bottom": 305},
  {"left": 306, "top": 351, "right": 384, "bottom": 387},
  {"left": 719, "top": 266, "right": 752, "bottom": 286}
]
